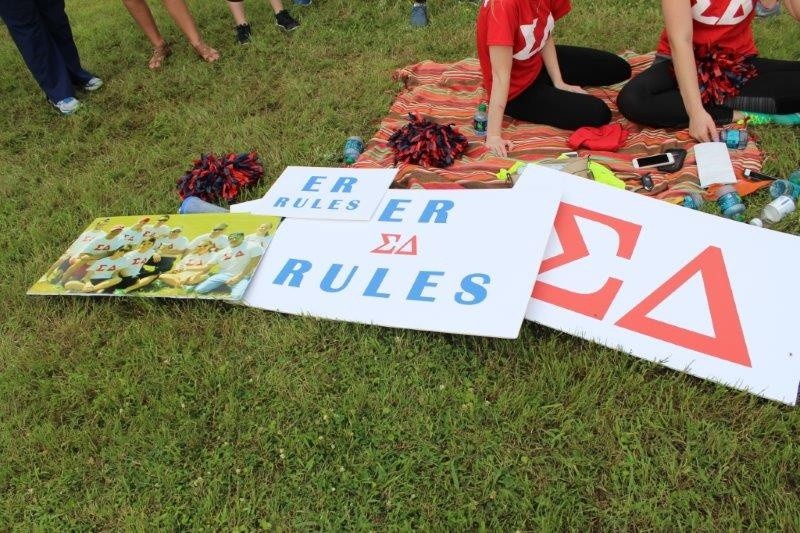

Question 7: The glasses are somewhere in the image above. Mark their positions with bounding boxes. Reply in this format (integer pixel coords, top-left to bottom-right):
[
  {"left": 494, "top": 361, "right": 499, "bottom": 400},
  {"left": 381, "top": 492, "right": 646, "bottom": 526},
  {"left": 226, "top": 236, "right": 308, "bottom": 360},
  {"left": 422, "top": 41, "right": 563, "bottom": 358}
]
[
  {"left": 213, "top": 228, "right": 224, "bottom": 232},
  {"left": 157, "top": 219, "right": 167, "bottom": 222},
  {"left": 197, "top": 245, "right": 212, "bottom": 247}
]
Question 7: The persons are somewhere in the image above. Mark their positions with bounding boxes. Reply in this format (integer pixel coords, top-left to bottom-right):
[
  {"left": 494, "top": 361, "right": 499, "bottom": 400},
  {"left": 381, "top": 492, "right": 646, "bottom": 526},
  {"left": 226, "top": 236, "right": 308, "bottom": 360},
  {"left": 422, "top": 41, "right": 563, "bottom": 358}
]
[
  {"left": 0, "top": 0, "right": 104, "bottom": 114},
  {"left": 410, "top": 0, "right": 427, "bottom": 27},
  {"left": 291, "top": 0, "right": 312, "bottom": 7},
  {"left": 477, "top": 0, "right": 632, "bottom": 158},
  {"left": 225, "top": 0, "right": 301, "bottom": 45},
  {"left": 122, "top": 0, "right": 220, "bottom": 70},
  {"left": 616, "top": 0, "right": 800, "bottom": 143}
]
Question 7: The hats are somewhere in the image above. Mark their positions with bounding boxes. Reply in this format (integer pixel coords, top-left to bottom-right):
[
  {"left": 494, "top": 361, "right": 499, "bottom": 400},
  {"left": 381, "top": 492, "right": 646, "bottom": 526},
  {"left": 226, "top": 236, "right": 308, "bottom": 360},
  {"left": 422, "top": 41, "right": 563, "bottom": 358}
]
[
  {"left": 171, "top": 224, "right": 183, "bottom": 232},
  {"left": 110, "top": 224, "right": 125, "bottom": 231}
]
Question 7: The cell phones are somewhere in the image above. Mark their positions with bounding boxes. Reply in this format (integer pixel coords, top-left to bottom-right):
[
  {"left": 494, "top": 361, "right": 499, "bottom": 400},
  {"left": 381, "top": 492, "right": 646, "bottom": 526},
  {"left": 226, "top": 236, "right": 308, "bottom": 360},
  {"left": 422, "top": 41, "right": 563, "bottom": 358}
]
[
  {"left": 659, "top": 148, "right": 687, "bottom": 173},
  {"left": 631, "top": 153, "right": 675, "bottom": 169},
  {"left": 744, "top": 167, "right": 780, "bottom": 182}
]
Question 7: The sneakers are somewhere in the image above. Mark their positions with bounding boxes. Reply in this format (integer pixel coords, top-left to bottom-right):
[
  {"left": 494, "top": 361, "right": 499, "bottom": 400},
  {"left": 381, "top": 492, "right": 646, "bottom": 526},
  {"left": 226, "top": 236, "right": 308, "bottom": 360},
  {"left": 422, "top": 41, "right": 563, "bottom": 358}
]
[
  {"left": 737, "top": 111, "right": 800, "bottom": 126},
  {"left": 274, "top": 9, "right": 300, "bottom": 32},
  {"left": 53, "top": 96, "right": 82, "bottom": 114},
  {"left": 85, "top": 76, "right": 104, "bottom": 90},
  {"left": 234, "top": 22, "right": 251, "bottom": 45}
]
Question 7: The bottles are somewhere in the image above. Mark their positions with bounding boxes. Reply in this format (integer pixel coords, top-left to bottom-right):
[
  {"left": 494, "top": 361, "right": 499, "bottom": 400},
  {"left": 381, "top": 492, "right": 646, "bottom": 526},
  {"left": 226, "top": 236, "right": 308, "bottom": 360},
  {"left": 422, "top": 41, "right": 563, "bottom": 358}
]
[
  {"left": 679, "top": 190, "right": 704, "bottom": 210},
  {"left": 473, "top": 104, "right": 488, "bottom": 136},
  {"left": 770, "top": 179, "right": 800, "bottom": 201},
  {"left": 342, "top": 136, "right": 365, "bottom": 166},
  {"left": 718, "top": 129, "right": 748, "bottom": 149},
  {"left": 750, "top": 194, "right": 796, "bottom": 228},
  {"left": 715, "top": 184, "right": 746, "bottom": 222},
  {"left": 788, "top": 168, "right": 800, "bottom": 185}
]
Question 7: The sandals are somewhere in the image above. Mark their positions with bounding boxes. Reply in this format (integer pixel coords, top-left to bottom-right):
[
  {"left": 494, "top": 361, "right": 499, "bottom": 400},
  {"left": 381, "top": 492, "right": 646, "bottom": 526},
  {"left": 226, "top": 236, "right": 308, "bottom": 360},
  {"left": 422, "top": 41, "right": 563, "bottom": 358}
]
[
  {"left": 190, "top": 39, "right": 220, "bottom": 62},
  {"left": 148, "top": 40, "right": 172, "bottom": 69}
]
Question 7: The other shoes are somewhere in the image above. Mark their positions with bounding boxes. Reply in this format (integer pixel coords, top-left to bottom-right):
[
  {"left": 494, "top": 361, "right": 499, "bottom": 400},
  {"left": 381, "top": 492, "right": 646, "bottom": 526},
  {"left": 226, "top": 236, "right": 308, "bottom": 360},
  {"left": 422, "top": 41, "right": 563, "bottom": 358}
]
[
  {"left": 296, "top": 0, "right": 312, "bottom": 7},
  {"left": 411, "top": 4, "right": 427, "bottom": 26},
  {"left": 113, "top": 288, "right": 127, "bottom": 295},
  {"left": 753, "top": 2, "right": 780, "bottom": 19}
]
